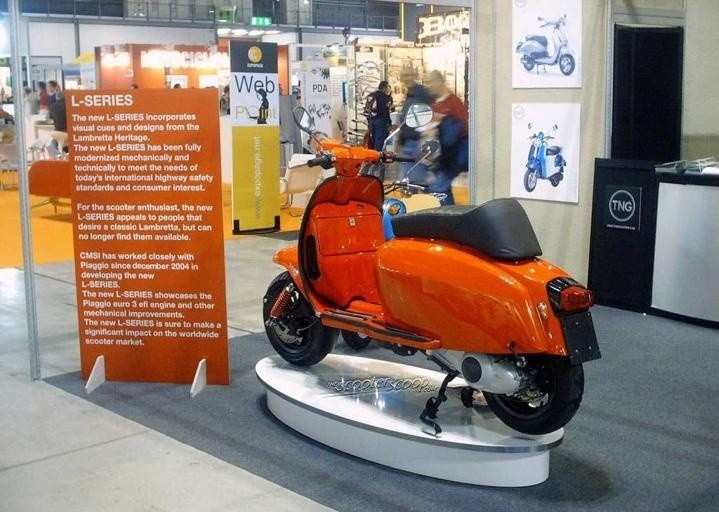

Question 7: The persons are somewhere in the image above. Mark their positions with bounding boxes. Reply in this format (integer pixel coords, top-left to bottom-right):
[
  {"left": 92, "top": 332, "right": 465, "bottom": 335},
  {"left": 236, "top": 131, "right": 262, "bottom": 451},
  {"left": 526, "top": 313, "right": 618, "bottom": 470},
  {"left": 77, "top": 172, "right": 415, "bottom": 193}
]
[
  {"left": 219, "top": 86, "right": 230, "bottom": 116},
  {"left": 0, "top": 80, "right": 69, "bottom": 152},
  {"left": 129, "top": 83, "right": 138, "bottom": 90},
  {"left": 364, "top": 81, "right": 394, "bottom": 151},
  {"left": 399, "top": 70, "right": 467, "bottom": 205},
  {"left": 173, "top": 84, "right": 182, "bottom": 88}
]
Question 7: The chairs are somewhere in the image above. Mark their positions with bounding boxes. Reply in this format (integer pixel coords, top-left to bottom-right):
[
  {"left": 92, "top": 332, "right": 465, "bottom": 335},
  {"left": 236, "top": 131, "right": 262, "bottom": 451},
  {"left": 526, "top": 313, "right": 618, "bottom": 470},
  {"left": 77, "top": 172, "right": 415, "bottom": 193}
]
[
  {"left": 26, "top": 161, "right": 72, "bottom": 213},
  {"left": 0, "top": 144, "right": 20, "bottom": 190},
  {"left": 279, "top": 154, "right": 322, "bottom": 208}
]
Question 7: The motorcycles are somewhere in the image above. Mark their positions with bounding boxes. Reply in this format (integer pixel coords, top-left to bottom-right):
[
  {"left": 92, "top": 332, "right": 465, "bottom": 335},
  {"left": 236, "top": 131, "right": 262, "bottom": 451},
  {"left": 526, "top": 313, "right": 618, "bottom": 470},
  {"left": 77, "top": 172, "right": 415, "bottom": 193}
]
[
  {"left": 262, "top": 104, "right": 602, "bottom": 441},
  {"left": 517, "top": 13, "right": 575, "bottom": 76},
  {"left": 341, "top": 135, "right": 449, "bottom": 357},
  {"left": 521, "top": 119, "right": 567, "bottom": 192}
]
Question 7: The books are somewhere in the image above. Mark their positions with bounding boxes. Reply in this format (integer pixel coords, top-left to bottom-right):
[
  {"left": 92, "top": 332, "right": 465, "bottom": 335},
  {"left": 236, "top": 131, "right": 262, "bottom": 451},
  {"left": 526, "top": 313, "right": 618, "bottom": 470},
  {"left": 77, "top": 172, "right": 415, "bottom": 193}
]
[{"left": 655, "top": 157, "right": 719, "bottom": 175}]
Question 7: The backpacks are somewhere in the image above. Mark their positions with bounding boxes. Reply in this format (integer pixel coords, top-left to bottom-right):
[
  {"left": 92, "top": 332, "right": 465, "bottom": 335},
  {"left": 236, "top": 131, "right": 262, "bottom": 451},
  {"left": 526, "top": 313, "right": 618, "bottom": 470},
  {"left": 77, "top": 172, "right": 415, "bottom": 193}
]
[{"left": 364, "top": 92, "right": 379, "bottom": 119}]
